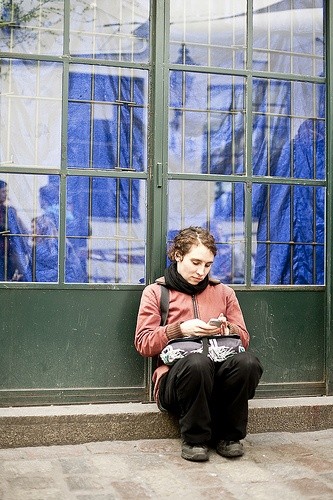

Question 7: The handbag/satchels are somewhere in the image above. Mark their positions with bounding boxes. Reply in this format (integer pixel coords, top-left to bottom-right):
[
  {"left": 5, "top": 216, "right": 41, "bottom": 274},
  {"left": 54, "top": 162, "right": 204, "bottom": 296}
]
[{"left": 159, "top": 334, "right": 241, "bottom": 366}]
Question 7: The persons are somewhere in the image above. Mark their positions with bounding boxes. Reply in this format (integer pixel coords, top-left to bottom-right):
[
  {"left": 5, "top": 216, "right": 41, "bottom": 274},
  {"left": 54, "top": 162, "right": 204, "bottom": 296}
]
[
  {"left": 0, "top": 179, "right": 89, "bottom": 283},
  {"left": 135, "top": 226, "right": 265, "bottom": 462},
  {"left": 167, "top": 221, "right": 255, "bottom": 284}
]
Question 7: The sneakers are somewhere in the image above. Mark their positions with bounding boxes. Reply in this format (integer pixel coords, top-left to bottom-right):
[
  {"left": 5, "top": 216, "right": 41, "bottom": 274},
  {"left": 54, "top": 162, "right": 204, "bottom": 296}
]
[
  {"left": 181, "top": 434, "right": 209, "bottom": 461},
  {"left": 216, "top": 440, "right": 244, "bottom": 457}
]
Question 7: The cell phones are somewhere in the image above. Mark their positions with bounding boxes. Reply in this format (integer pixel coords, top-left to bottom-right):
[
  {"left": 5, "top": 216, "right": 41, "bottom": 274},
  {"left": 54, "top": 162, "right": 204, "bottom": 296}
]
[{"left": 207, "top": 318, "right": 223, "bottom": 328}]
